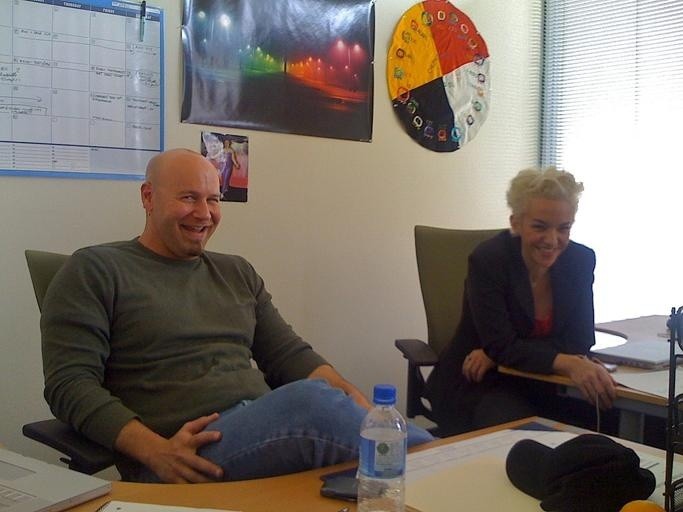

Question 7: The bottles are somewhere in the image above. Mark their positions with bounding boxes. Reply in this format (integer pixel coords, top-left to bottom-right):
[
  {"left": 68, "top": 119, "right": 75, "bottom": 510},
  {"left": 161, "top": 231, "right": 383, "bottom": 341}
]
[{"left": 357, "top": 384, "right": 407, "bottom": 512}]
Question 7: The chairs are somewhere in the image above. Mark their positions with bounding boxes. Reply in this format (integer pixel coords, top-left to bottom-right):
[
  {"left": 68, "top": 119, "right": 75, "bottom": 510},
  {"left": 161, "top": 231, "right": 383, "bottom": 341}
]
[
  {"left": 21, "top": 247, "right": 116, "bottom": 474},
  {"left": 396, "top": 225, "right": 513, "bottom": 427}
]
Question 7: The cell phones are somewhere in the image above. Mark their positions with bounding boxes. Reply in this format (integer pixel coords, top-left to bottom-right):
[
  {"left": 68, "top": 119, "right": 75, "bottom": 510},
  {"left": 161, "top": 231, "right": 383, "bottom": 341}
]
[{"left": 320, "top": 475, "right": 389, "bottom": 502}]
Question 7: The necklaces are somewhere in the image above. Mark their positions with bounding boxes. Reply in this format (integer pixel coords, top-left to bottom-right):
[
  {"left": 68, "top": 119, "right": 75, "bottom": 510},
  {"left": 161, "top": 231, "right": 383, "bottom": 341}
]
[{"left": 530, "top": 281, "right": 537, "bottom": 289}]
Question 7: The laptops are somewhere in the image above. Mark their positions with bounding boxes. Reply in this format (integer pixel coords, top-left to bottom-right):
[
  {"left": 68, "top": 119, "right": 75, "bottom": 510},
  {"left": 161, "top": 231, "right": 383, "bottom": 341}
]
[{"left": 0, "top": 444, "right": 112, "bottom": 512}]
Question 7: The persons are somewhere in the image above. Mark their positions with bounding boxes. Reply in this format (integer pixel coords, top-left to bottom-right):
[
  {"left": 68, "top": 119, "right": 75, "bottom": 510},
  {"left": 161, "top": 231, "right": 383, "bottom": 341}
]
[
  {"left": 211, "top": 139, "right": 240, "bottom": 195},
  {"left": 418, "top": 162, "right": 620, "bottom": 436},
  {"left": 35, "top": 148, "right": 435, "bottom": 485}
]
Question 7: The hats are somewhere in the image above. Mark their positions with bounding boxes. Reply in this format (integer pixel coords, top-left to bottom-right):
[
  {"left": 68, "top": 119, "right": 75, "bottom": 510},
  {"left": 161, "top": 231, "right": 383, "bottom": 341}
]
[{"left": 503, "top": 432, "right": 657, "bottom": 512}]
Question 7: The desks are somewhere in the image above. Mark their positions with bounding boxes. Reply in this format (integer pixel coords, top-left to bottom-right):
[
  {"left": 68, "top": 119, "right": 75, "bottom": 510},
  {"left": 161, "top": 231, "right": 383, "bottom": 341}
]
[{"left": 59, "top": 316, "right": 683, "bottom": 512}]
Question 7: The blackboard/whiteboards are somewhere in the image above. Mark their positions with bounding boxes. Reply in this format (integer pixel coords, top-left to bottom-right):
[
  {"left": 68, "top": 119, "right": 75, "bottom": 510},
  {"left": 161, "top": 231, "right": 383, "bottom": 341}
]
[{"left": 2, "top": 0, "right": 166, "bottom": 180}]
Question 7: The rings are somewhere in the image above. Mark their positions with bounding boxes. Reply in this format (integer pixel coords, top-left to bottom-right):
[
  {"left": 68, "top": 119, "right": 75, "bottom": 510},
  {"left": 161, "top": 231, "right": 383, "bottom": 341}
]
[{"left": 466, "top": 355, "right": 470, "bottom": 360}]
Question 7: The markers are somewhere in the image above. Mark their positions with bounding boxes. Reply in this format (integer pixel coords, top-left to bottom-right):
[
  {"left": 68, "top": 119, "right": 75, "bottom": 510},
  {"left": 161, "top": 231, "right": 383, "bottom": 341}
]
[{"left": 141, "top": 1, "right": 147, "bottom": 41}]
